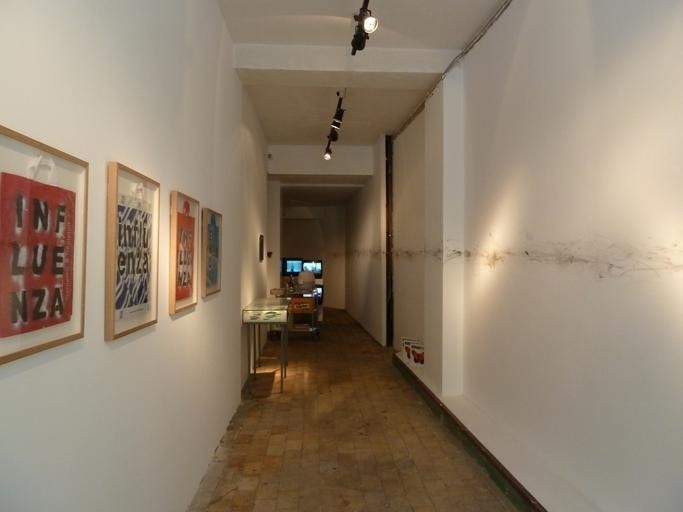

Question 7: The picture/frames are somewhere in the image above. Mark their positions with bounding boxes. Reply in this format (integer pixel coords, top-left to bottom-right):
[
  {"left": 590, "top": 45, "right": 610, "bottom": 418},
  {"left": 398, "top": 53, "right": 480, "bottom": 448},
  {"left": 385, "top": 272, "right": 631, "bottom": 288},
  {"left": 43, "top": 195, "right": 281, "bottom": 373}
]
[
  {"left": 201, "top": 208, "right": 222, "bottom": 299},
  {"left": 104, "top": 161, "right": 161, "bottom": 341},
  {"left": 0, "top": 124, "right": 89, "bottom": 365},
  {"left": 169, "top": 191, "right": 200, "bottom": 315}
]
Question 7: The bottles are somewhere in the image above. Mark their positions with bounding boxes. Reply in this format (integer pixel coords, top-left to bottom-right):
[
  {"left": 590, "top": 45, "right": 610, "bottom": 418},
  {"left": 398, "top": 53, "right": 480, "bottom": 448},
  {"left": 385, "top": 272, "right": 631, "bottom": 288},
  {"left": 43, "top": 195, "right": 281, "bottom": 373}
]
[{"left": 288, "top": 274, "right": 294, "bottom": 292}]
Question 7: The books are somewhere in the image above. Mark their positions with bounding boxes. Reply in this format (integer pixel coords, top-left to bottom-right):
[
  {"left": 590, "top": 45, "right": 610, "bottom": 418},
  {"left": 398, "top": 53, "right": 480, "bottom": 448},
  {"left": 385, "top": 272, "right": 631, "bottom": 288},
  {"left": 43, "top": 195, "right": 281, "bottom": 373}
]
[{"left": 400, "top": 336, "right": 424, "bottom": 369}]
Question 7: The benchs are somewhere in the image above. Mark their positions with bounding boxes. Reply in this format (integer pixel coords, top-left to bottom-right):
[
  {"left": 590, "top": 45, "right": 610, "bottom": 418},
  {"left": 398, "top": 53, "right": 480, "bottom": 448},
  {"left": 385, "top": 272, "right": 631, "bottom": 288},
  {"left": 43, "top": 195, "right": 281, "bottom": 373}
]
[{"left": 267, "top": 297, "right": 319, "bottom": 343}]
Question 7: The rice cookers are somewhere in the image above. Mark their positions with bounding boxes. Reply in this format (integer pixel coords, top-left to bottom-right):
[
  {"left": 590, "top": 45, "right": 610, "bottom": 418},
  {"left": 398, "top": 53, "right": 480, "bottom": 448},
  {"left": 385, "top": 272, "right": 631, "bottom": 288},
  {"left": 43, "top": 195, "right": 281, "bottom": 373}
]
[{"left": 295, "top": 270, "right": 315, "bottom": 292}]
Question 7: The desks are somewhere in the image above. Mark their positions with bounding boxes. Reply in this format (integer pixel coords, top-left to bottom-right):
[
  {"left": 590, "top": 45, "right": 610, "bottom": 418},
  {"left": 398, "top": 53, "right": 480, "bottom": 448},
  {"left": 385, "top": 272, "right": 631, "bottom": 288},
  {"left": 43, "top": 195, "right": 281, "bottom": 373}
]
[
  {"left": 271, "top": 287, "right": 313, "bottom": 297},
  {"left": 243, "top": 297, "right": 291, "bottom": 393}
]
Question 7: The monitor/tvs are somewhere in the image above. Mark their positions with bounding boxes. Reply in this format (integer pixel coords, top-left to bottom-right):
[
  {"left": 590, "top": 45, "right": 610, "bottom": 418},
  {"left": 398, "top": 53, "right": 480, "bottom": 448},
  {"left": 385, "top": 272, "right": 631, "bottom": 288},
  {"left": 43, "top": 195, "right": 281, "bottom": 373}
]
[
  {"left": 282, "top": 257, "right": 302, "bottom": 276},
  {"left": 302, "top": 260, "right": 322, "bottom": 279},
  {"left": 301, "top": 285, "right": 323, "bottom": 304}
]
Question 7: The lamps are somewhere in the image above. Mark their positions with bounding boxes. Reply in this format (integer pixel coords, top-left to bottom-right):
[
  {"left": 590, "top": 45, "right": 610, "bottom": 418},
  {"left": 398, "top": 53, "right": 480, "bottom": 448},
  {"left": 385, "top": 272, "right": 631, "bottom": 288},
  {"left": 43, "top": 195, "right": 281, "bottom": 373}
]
[
  {"left": 351, "top": 0, "right": 378, "bottom": 56},
  {"left": 323, "top": 97, "right": 345, "bottom": 160}
]
